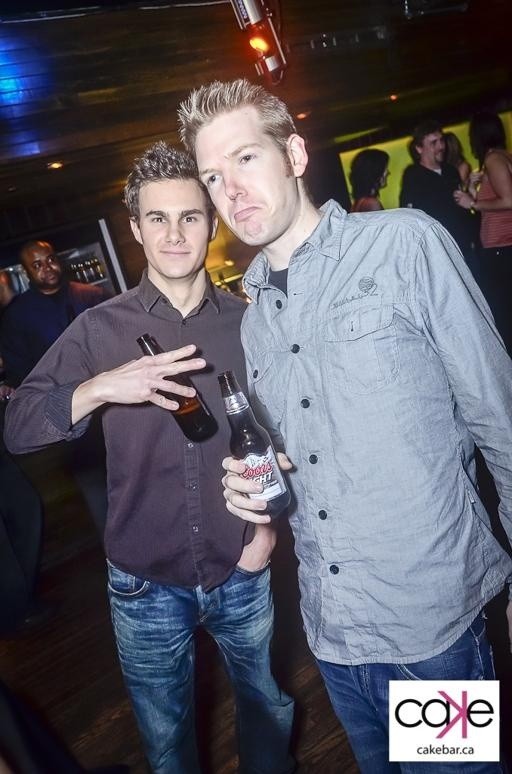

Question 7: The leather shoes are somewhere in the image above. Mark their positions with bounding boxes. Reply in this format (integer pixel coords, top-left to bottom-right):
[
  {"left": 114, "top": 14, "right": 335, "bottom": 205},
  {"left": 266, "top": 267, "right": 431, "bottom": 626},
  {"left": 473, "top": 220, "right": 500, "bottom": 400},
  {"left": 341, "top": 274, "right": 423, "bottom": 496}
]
[{"left": 0, "top": 605, "right": 65, "bottom": 632}]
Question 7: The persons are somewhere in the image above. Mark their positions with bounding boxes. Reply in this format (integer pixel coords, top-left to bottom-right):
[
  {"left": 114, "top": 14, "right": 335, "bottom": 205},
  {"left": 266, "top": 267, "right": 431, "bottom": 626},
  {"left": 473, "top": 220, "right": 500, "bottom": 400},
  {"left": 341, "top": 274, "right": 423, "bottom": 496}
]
[
  {"left": 443, "top": 131, "right": 471, "bottom": 186},
  {"left": 346, "top": 149, "right": 390, "bottom": 212},
  {"left": 0, "top": 356, "right": 54, "bottom": 630},
  {"left": 400, "top": 119, "right": 484, "bottom": 295},
  {"left": 176, "top": 76, "right": 512, "bottom": 773},
  {"left": 0, "top": 240, "right": 114, "bottom": 546},
  {"left": 2, "top": 140, "right": 298, "bottom": 771},
  {"left": 451, "top": 113, "right": 512, "bottom": 361}
]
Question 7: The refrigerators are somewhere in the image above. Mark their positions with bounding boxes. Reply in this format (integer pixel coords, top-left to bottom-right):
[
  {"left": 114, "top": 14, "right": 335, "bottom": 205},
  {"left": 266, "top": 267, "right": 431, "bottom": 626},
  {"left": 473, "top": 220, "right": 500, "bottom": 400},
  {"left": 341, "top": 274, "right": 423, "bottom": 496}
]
[{"left": 0, "top": 216, "right": 128, "bottom": 302}]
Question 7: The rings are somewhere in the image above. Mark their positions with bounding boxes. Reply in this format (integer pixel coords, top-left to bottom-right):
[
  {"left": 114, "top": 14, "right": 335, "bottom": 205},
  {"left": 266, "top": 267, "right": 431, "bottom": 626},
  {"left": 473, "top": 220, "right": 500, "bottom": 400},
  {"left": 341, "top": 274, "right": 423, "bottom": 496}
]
[{"left": 223, "top": 474, "right": 237, "bottom": 489}]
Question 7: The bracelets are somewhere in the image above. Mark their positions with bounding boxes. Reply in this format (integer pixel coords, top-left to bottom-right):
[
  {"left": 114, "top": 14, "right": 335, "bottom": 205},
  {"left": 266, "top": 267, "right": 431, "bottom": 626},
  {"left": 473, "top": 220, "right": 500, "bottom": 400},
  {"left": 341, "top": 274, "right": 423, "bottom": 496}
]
[{"left": 469, "top": 198, "right": 478, "bottom": 210}]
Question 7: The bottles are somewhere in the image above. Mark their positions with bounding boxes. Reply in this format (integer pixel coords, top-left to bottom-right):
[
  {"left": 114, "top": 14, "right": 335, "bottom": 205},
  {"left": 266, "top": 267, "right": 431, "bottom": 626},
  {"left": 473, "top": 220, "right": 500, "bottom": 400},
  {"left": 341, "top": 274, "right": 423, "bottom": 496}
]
[
  {"left": 135, "top": 331, "right": 220, "bottom": 444},
  {"left": 68, "top": 258, "right": 106, "bottom": 285},
  {"left": 217, "top": 368, "right": 293, "bottom": 518}
]
